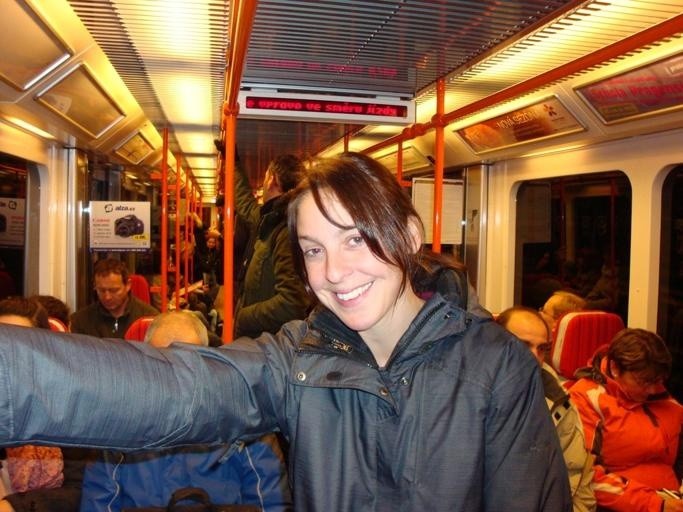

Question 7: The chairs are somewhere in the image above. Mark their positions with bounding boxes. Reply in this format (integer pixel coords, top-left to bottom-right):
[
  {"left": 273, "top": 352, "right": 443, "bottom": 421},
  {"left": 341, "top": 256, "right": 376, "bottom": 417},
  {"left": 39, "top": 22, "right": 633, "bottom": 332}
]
[
  {"left": 549, "top": 310, "right": 625, "bottom": 391},
  {"left": 45, "top": 316, "right": 68, "bottom": 333},
  {"left": 128, "top": 275, "right": 150, "bottom": 308},
  {"left": 122, "top": 317, "right": 153, "bottom": 341}
]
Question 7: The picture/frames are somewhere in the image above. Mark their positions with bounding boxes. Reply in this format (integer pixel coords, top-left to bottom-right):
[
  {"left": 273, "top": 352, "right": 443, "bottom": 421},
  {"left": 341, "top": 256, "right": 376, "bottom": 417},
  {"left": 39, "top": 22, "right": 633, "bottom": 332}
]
[
  {"left": 111, "top": 130, "right": 155, "bottom": 167},
  {"left": 33, "top": 61, "right": 127, "bottom": 140},
  {"left": 410, "top": 178, "right": 465, "bottom": 246},
  {"left": 0, "top": 0, "right": 74, "bottom": 91},
  {"left": 571, "top": 49, "right": 683, "bottom": 127},
  {"left": 452, "top": 93, "right": 588, "bottom": 156},
  {"left": 373, "top": 144, "right": 433, "bottom": 176}
]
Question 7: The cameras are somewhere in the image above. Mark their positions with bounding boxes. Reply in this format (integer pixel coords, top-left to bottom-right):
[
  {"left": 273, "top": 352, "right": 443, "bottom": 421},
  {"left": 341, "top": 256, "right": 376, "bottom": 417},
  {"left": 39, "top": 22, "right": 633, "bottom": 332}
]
[{"left": 115, "top": 215, "right": 143, "bottom": 237}]
